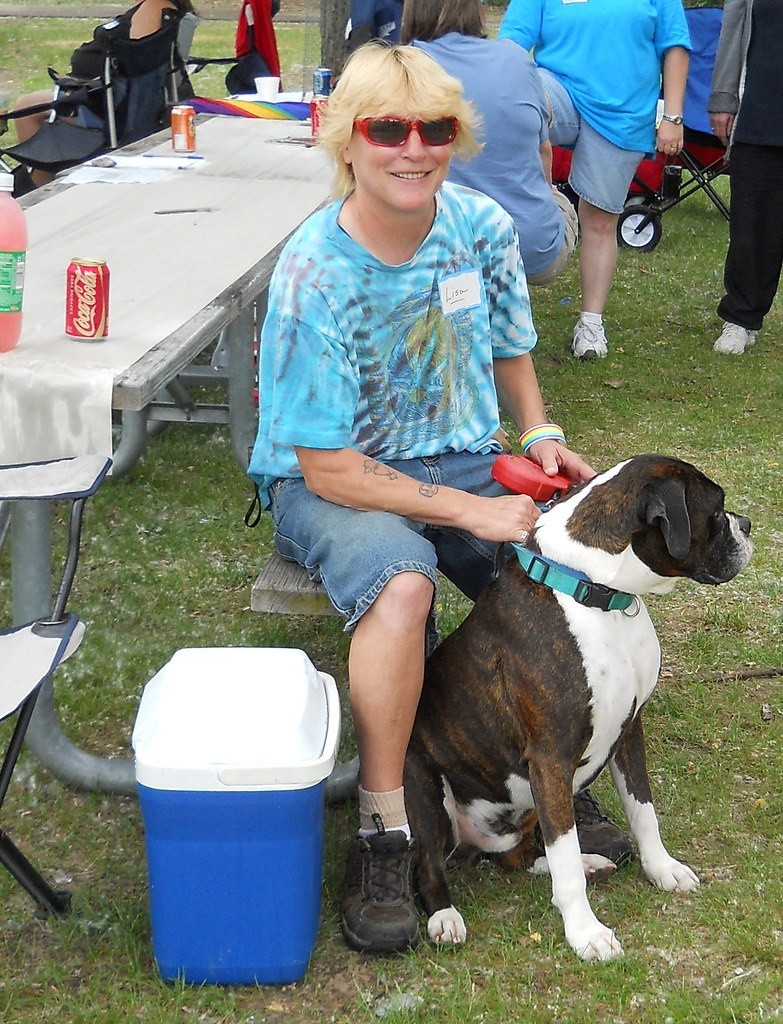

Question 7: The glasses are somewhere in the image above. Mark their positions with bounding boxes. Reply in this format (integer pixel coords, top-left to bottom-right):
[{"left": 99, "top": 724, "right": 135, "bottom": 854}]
[{"left": 353, "top": 117, "right": 459, "bottom": 146}]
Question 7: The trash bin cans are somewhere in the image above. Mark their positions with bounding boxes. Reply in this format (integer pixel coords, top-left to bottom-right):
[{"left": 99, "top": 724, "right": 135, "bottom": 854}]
[{"left": 132, "top": 647, "right": 341, "bottom": 986}]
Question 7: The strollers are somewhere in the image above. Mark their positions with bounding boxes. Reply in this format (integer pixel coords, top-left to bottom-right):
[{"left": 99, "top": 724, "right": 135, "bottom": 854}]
[{"left": 553, "top": 4, "right": 731, "bottom": 255}]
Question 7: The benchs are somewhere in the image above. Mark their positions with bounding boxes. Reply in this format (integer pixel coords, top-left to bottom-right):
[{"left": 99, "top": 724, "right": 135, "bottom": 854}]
[{"left": 249, "top": 550, "right": 350, "bottom": 618}]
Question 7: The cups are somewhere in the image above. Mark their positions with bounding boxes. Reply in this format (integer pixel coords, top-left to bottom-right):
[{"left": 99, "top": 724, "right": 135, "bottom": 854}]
[{"left": 254, "top": 76, "right": 279, "bottom": 93}]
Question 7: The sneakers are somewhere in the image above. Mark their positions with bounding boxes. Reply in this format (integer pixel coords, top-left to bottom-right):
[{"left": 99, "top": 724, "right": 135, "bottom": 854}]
[
  {"left": 571, "top": 788, "right": 631, "bottom": 864},
  {"left": 341, "top": 825, "right": 418, "bottom": 955},
  {"left": 713, "top": 321, "right": 758, "bottom": 355},
  {"left": 570, "top": 317, "right": 607, "bottom": 361}
]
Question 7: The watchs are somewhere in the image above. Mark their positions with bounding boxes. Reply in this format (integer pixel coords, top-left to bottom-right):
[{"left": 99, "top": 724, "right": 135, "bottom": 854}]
[{"left": 662, "top": 114, "right": 683, "bottom": 126}]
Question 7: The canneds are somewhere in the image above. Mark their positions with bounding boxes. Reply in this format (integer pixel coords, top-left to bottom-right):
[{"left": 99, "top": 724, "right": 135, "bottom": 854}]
[
  {"left": 170, "top": 105, "right": 196, "bottom": 152},
  {"left": 64, "top": 257, "right": 110, "bottom": 342},
  {"left": 313, "top": 68, "right": 333, "bottom": 97},
  {"left": 309, "top": 95, "right": 329, "bottom": 137}
]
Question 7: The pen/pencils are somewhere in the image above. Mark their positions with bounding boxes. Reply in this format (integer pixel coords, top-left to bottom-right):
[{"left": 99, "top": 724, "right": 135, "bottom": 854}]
[
  {"left": 143, "top": 154, "right": 204, "bottom": 159},
  {"left": 154, "top": 207, "right": 211, "bottom": 214}
]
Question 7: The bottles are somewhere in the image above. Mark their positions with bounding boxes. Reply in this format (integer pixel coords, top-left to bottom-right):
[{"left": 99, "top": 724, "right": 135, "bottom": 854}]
[{"left": 0, "top": 173, "right": 27, "bottom": 352}]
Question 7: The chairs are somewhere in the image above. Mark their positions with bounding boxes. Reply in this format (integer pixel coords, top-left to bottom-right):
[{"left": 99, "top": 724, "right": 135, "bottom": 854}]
[
  {"left": 0, "top": 8, "right": 200, "bottom": 200},
  {"left": 0, "top": 453, "right": 115, "bottom": 923}
]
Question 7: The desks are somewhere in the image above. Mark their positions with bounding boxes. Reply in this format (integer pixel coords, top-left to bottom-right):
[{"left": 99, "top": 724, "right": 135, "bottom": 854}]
[{"left": 0, "top": 90, "right": 335, "bottom": 795}]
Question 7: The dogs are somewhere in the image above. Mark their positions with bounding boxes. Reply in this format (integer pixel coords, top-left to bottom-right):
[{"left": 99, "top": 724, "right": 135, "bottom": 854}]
[{"left": 401, "top": 452, "right": 753, "bottom": 962}]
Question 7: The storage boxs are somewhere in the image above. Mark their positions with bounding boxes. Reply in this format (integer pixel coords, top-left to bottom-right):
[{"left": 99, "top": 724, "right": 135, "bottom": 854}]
[{"left": 130, "top": 647, "right": 342, "bottom": 985}]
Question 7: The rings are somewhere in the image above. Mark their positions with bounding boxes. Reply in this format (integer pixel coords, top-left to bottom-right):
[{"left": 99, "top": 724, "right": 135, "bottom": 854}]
[
  {"left": 710, "top": 127, "right": 715, "bottom": 131},
  {"left": 671, "top": 145, "right": 677, "bottom": 148}
]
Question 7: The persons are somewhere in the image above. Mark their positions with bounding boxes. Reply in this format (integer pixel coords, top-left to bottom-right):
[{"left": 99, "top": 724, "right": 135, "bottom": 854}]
[
  {"left": 707, "top": 0, "right": 783, "bottom": 354},
  {"left": 393, "top": 0, "right": 578, "bottom": 284},
  {"left": 249, "top": 48, "right": 637, "bottom": 954},
  {"left": 495, "top": 0, "right": 693, "bottom": 353},
  {"left": 12, "top": 1, "right": 199, "bottom": 188},
  {"left": 345, "top": 0, "right": 403, "bottom": 50}
]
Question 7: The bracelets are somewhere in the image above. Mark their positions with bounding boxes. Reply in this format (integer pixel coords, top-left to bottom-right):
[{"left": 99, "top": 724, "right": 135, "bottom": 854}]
[{"left": 518, "top": 423, "right": 567, "bottom": 454}]
